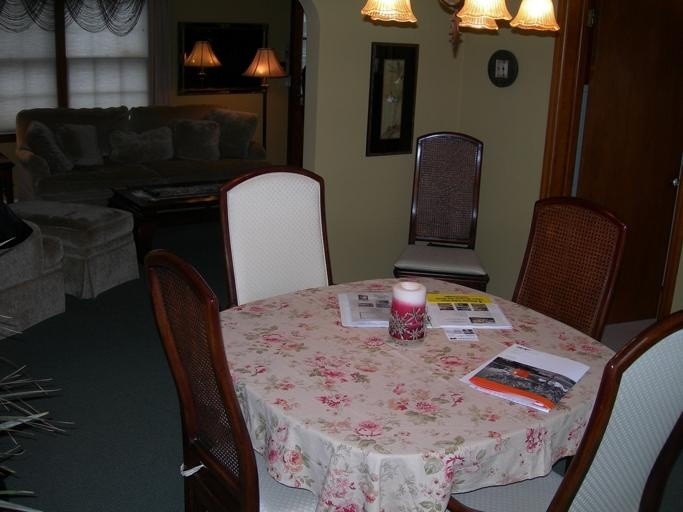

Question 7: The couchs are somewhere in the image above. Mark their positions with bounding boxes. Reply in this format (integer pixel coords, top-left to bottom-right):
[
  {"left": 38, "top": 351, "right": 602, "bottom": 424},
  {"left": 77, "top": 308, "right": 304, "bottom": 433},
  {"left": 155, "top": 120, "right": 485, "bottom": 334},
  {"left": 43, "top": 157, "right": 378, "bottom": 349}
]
[{"left": 12, "top": 103, "right": 271, "bottom": 206}]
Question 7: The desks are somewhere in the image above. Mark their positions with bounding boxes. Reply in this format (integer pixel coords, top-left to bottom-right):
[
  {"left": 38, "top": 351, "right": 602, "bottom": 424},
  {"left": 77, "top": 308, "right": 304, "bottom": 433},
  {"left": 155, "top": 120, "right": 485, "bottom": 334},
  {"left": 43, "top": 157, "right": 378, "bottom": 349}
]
[{"left": 217, "top": 278, "right": 616, "bottom": 512}]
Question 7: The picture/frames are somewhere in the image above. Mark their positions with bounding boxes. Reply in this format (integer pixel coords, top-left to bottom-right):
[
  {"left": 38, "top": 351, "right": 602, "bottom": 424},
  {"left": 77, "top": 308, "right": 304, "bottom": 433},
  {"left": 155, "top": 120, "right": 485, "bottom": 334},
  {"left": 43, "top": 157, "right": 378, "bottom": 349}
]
[{"left": 364, "top": 43, "right": 421, "bottom": 157}]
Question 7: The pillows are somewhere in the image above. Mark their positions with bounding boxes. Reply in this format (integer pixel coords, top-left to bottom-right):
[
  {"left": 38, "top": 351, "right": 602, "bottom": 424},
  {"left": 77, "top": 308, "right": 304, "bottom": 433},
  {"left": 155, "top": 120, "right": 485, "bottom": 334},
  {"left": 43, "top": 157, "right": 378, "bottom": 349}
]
[{"left": 23, "top": 108, "right": 257, "bottom": 174}]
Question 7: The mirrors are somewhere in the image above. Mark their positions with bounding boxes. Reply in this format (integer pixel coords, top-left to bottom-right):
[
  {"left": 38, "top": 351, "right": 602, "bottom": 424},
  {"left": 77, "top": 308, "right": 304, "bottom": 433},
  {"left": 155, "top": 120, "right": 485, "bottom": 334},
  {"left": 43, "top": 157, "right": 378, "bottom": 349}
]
[{"left": 175, "top": 21, "right": 268, "bottom": 95}]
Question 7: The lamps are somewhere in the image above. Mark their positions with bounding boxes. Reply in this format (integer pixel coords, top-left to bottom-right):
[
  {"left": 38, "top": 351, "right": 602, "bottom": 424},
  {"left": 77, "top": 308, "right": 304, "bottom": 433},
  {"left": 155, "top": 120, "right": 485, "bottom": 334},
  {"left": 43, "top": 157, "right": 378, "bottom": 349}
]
[
  {"left": 360, "top": 0, "right": 560, "bottom": 33},
  {"left": 181, "top": 41, "right": 222, "bottom": 86},
  {"left": 240, "top": 46, "right": 289, "bottom": 150}
]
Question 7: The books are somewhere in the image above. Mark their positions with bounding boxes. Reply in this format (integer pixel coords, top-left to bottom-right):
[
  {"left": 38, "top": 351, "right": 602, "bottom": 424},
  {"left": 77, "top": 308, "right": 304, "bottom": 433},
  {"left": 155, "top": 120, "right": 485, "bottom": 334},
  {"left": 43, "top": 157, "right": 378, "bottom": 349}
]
[
  {"left": 456, "top": 342, "right": 591, "bottom": 413},
  {"left": 336, "top": 290, "right": 513, "bottom": 330}
]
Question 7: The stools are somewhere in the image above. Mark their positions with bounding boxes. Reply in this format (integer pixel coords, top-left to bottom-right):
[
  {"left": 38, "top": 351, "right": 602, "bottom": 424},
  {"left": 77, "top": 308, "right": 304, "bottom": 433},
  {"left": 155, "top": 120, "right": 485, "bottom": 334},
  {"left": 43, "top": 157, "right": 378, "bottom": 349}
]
[{"left": 7, "top": 201, "right": 140, "bottom": 301}]
